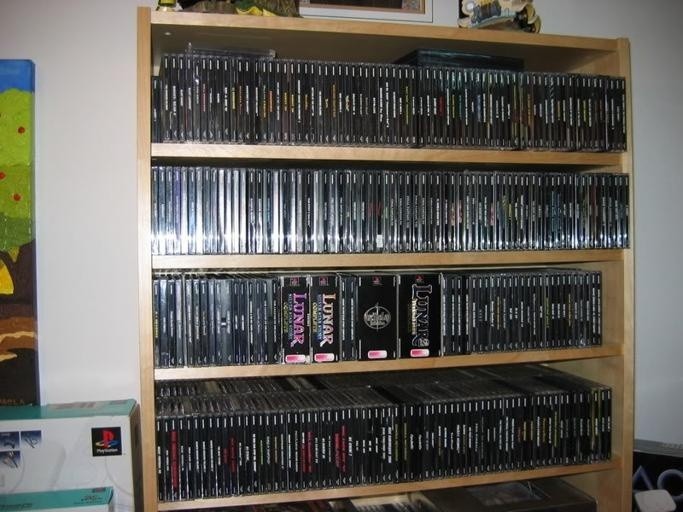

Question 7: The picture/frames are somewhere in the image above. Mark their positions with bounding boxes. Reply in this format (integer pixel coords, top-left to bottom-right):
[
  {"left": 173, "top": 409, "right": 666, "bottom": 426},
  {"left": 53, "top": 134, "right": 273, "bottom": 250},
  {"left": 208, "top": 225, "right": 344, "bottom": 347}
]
[{"left": 300, "top": 0, "right": 434, "bottom": 23}]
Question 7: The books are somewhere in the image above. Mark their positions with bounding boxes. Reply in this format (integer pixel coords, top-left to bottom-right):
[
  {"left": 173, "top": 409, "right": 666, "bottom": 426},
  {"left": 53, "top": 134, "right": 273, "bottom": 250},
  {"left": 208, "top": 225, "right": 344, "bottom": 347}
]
[
  {"left": 152, "top": 268, "right": 603, "bottom": 369},
  {"left": 150, "top": 166, "right": 629, "bottom": 255},
  {"left": 151, "top": 49, "right": 626, "bottom": 154},
  {"left": 155, "top": 362, "right": 613, "bottom": 511}
]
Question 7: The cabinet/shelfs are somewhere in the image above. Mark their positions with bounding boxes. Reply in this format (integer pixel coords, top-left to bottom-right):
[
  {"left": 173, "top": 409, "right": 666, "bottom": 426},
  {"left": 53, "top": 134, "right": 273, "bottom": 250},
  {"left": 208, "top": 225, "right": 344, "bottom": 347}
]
[{"left": 137, "top": 6, "right": 636, "bottom": 512}]
